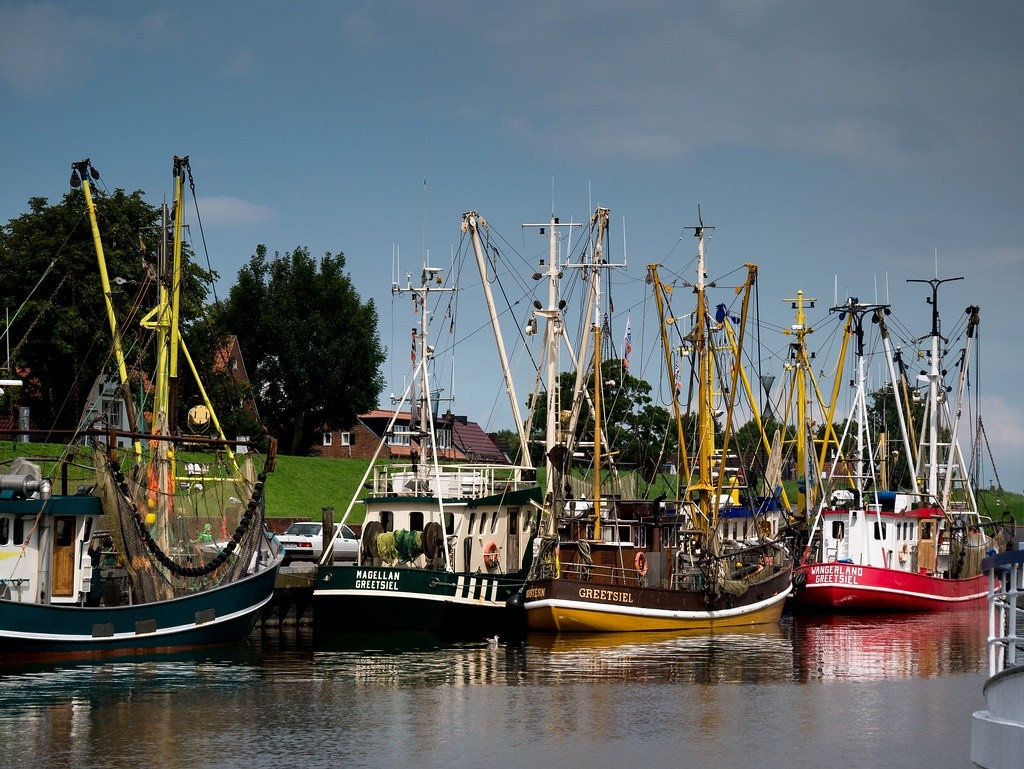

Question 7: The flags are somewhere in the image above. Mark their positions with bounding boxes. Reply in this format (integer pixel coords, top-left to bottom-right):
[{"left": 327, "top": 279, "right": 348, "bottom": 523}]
[
  {"left": 674, "top": 362, "right": 681, "bottom": 397},
  {"left": 624, "top": 313, "right": 634, "bottom": 373}
]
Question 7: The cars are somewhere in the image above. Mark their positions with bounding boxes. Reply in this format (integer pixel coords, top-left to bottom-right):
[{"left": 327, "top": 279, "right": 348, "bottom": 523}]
[{"left": 273, "top": 521, "right": 364, "bottom": 566}]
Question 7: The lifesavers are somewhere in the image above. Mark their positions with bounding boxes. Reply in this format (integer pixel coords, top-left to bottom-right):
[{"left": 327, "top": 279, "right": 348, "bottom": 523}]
[
  {"left": 634, "top": 551, "right": 651, "bottom": 578},
  {"left": 899, "top": 543, "right": 911, "bottom": 565},
  {"left": 484, "top": 542, "right": 499, "bottom": 570}
]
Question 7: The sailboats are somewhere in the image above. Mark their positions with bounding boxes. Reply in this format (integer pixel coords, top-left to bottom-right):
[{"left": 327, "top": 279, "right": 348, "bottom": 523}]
[
  {"left": 0, "top": 150, "right": 290, "bottom": 668},
  {"left": 311, "top": 200, "right": 1006, "bottom": 634}
]
